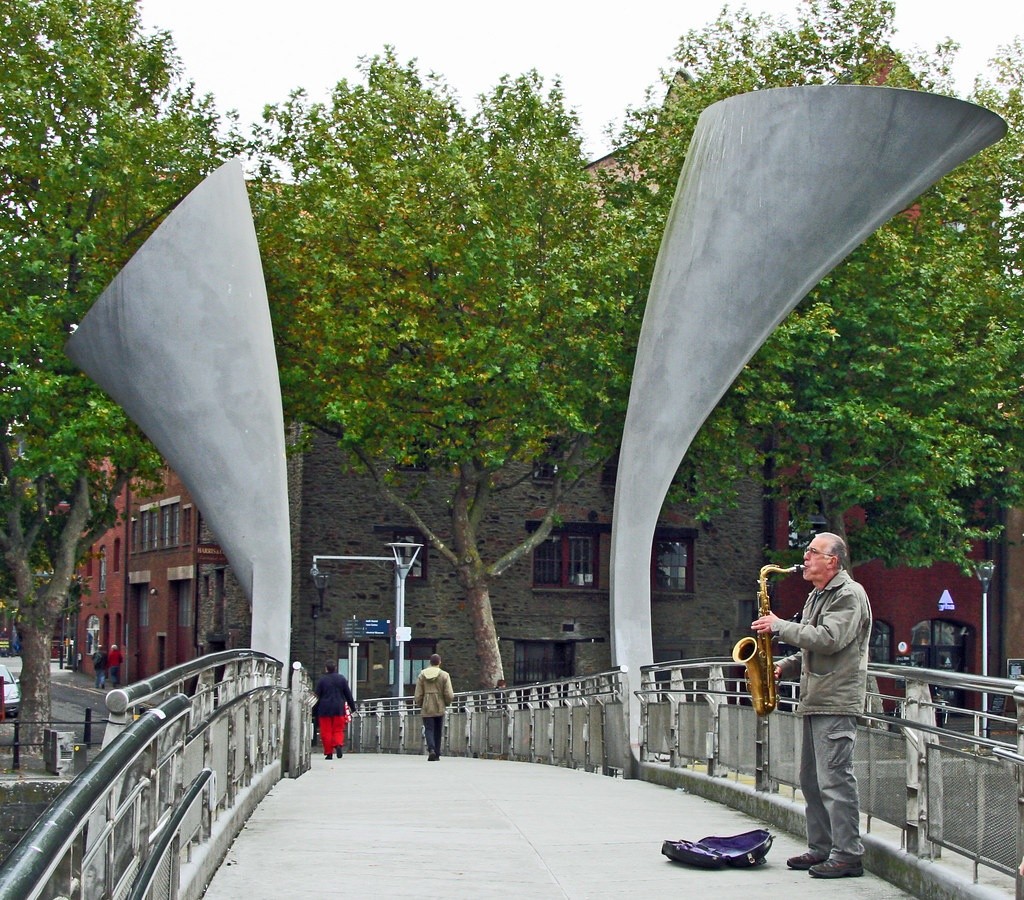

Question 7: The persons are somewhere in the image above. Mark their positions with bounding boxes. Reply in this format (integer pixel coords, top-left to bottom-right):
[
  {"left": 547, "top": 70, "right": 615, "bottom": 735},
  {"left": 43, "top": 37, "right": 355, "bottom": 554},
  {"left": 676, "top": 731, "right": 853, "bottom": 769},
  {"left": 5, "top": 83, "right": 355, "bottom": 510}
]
[
  {"left": 751, "top": 532, "right": 873, "bottom": 877},
  {"left": 415, "top": 653, "right": 454, "bottom": 761},
  {"left": 88, "top": 633, "right": 124, "bottom": 690},
  {"left": 495, "top": 680, "right": 507, "bottom": 712},
  {"left": 315, "top": 658, "right": 357, "bottom": 760}
]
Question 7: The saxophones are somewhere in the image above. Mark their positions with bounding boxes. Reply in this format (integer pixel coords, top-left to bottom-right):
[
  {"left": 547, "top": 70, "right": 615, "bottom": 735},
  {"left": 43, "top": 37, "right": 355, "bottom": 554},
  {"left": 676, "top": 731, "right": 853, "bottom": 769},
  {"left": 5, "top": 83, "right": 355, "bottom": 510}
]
[{"left": 732, "top": 564, "right": 807, "bottom": 716}]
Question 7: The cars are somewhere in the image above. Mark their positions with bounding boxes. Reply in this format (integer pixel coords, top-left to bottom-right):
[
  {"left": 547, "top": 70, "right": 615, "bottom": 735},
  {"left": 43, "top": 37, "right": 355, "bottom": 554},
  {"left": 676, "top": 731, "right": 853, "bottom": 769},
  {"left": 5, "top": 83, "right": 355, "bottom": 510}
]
[{"left": 0, "top": 664, "right": 20, "bottom": 717}]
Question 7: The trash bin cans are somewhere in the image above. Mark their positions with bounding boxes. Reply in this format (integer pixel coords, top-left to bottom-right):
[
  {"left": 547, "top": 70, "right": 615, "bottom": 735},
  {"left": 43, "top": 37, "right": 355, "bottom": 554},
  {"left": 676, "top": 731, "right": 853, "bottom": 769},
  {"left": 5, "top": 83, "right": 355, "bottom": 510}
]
[{"left": 934, "top": 695, "right": 949, "bottom": 727}]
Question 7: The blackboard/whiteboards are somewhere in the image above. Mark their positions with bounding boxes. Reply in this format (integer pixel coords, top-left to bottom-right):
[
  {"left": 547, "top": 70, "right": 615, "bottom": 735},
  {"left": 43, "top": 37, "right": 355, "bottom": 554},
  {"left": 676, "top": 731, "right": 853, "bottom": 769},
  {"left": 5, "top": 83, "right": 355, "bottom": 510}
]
[
  {"left": 894, "top": 654, "right": 915, "bottom": 691},
  {"left": 989, "top": 692, "right": 1007, "bottom": 714}
]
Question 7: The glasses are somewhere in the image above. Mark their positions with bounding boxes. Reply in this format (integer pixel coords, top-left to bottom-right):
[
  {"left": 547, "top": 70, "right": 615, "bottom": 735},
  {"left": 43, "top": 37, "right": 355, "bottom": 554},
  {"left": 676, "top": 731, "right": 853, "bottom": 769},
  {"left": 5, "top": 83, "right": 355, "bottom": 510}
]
[{"left": 803, "top": 547, "right": 840, "bottom": 563}]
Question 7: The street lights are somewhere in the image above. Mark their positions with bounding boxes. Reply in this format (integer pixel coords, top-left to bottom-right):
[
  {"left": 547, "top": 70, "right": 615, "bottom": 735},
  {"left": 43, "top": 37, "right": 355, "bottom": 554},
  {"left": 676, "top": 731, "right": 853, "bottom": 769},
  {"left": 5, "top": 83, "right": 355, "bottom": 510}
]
[
  {"left": 383, "top": 541, "right": 425, "bottom": 710},
  {"left": 973, "top": 560, "right": 997, "bottom": 737}
]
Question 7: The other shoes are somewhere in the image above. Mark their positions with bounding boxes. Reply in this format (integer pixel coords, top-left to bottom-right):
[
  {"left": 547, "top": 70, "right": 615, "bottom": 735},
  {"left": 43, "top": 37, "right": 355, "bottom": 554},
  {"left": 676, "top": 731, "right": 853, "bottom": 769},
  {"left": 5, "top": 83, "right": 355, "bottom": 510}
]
[
  {"left": 325, "top": 755, "right": 332, "bottom": 760},
  {"left": 428, "top": 752, "right": 440, "bottom": 761},
  {"left": 337, "top": 746, "right": 343, "bottom": 758}
]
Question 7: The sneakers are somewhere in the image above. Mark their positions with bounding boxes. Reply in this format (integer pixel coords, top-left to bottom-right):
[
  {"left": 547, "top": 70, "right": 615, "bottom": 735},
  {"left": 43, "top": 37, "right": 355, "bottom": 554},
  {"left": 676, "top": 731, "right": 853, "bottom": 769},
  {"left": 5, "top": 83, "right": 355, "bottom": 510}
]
[
  {"left": 786, "top": 853, "right": 826, "bottom": 869},
  {"left": 809, "top": 859, "right": 864, "bottom": 878}
]
